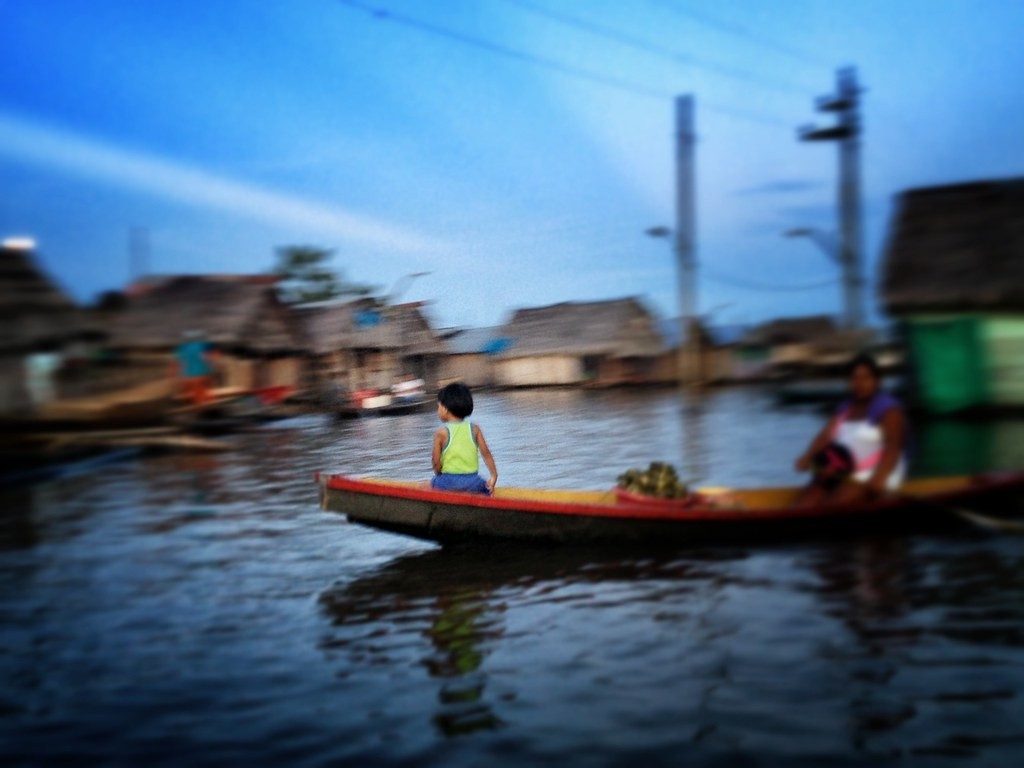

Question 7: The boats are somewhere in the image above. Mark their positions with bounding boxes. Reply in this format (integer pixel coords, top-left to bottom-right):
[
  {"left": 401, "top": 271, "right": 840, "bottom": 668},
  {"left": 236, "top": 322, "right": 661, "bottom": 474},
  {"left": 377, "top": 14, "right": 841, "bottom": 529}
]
[
  {"left": 168, "top": 394, "right": 321, "bottom": 432},
  {"left": 328, "top": 379, "right": 438, "bottom": 415},
  {"left": 311, "top": 467, "right": 1020, "bottom": 549}
]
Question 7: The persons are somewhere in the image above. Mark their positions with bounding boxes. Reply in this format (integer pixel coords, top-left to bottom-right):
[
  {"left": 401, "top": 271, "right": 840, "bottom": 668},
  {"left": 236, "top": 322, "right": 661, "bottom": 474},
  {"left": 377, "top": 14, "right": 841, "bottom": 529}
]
[
  {"left": 431, "top": 383, "right": 497, "bottom": 497},
  {"left": 177, "top": 329, "right": 212, "bottom": 377},
  {"left": 794, "top": 352, "right": 914, "bottom": 508}
]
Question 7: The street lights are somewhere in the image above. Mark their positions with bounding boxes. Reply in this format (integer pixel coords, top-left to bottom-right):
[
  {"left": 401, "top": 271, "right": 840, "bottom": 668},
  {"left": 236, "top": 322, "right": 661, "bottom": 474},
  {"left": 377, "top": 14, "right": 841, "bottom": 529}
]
[
  {"left": 644, "top": 225, "right": 700, "bottom": 413},
  {"left": 781, "top": 227, "right": 865, "bottom": 328}
]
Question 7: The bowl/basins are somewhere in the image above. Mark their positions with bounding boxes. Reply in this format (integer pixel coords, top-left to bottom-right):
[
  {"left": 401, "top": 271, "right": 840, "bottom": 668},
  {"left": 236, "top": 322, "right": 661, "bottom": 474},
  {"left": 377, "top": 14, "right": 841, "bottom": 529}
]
[{"left": 614, "top": 484, "right": 693, "bottom": 508}]
